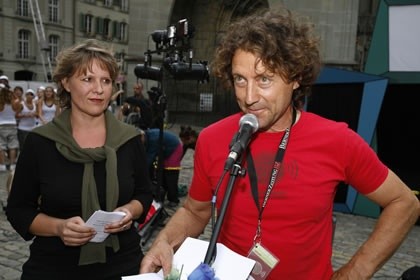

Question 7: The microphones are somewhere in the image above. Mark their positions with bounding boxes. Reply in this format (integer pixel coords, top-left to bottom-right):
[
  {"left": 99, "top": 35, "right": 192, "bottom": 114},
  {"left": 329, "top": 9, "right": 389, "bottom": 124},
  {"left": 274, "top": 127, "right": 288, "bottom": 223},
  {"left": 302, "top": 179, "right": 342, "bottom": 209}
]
[{"left": 224, "top": 114, "right": 258, "bottom": 171}]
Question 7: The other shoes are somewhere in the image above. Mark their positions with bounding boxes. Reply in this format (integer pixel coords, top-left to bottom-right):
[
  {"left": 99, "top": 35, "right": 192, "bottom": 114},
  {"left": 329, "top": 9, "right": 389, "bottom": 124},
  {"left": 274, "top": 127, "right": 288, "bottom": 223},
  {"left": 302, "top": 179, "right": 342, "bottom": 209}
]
[{"left": 0, "top": 165, "right": 17, "bottom": 172}]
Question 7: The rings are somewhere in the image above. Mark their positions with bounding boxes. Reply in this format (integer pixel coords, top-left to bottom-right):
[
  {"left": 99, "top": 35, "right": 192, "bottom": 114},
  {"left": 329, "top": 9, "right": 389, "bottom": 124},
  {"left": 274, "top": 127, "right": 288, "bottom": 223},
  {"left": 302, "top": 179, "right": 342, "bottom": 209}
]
[{"left": 123, "top": 225, "right": 128, "bottom": 231}]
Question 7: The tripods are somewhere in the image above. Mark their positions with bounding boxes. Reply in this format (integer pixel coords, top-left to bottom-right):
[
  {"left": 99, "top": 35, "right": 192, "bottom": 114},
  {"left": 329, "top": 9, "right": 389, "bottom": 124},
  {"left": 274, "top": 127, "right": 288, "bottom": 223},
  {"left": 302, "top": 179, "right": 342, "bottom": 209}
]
[{"left": 140, "top": 60, "right": 173, "bottom": 246}]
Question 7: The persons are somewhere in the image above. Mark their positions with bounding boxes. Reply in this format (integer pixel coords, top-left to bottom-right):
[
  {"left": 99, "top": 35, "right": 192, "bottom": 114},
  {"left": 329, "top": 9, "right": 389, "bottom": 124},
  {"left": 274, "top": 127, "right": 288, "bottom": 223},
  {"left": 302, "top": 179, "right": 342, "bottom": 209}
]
[
  {"left": 138, "top": 6, "right": 420, "bottom": 280},
  {"left": 0, "top": 75, "right": 67, "bottom": 209},
  {"left": 110, "top": 68, "right": 199, "bottom": 208},
  {"left": 5, "top": 37, "right": 154, "bottom": 280}
]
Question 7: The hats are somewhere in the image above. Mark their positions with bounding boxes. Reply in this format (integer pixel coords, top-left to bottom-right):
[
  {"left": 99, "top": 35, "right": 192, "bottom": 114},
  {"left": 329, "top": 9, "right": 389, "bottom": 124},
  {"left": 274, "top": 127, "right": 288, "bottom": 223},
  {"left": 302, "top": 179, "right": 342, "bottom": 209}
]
[
  {"left": 0, "top": 75, "right": 9, "bottom": 81},
  {"left": 53, "top": 90, "right": 58, "bottom": 94},
  {"left": 38, "top": 86, "right": 45, "bottom": 91},
  {"left": 24, "top": 89, "right": 35, "bottom": 96}
]
